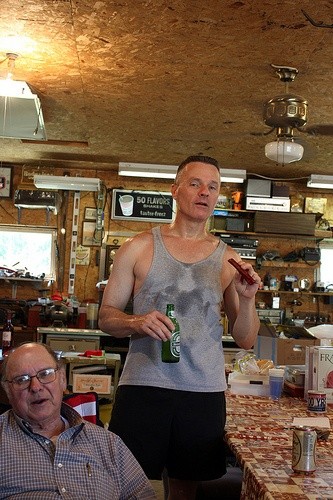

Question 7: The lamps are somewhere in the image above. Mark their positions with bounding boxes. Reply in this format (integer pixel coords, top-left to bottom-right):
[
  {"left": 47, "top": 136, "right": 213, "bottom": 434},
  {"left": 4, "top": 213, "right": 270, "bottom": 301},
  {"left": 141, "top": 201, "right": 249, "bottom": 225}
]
[
  {"left": 307, "top": 174, "right": 333, "bottom": 189},
  {"left": 14, "top": 189, "right": 55, "bottom": 209},
  {"left": 0, "top": 53, "right": 48, "bottom": 142},
  {"left": 118, "top": 161, "right": 246, "bottom": 183},
  {"left": 33, "top": 175, "right": 100, "bottom": 191},
  {"left": 262, "top": 64, "right": 308, "bottom": 168}
]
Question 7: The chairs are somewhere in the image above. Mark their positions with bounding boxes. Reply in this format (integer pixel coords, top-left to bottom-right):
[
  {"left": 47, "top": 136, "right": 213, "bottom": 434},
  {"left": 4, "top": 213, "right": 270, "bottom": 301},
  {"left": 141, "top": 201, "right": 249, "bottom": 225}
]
[{"left": 62, "top": 392, "right": 105, "bottom": 427}]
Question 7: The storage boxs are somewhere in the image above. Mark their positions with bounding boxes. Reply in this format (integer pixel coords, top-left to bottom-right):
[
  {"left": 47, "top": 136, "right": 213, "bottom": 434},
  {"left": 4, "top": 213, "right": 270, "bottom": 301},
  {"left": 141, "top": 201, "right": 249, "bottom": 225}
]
[
  {"left": 253, "top": 211, "right": 316, "bottom": 234},
  {"left": 246, "top": 197, "right": 290, "bottom": 212},
  {"left": 304, "top": 197, "right": 327, "bottom": 214},
  {"left": 291, "top": 196, "right": 304, "bottom": 213},
  {"left": 245, "top": 178, "right": 272, "bottom": 197},
  {"left": 272, "top": 186, "right": 289, "bottom": 197},
  {"left": 228, "top": 346, "right": 333, "bottom": 404},
  {"left": 37, "top": 325, "right": 121, "bottom": 427},
  {"left": 226, "top": 218, "right": 245, "bottom": 231}
]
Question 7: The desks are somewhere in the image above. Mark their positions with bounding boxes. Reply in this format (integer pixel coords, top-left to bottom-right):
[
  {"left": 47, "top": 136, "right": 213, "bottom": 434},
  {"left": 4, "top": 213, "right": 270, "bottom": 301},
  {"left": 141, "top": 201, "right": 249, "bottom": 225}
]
[
  {"left": 0, "top": 277, "right": 43, "bottom": 299},
  {"left": 224, "top": 364, "right": 333, "bottom": 500}
]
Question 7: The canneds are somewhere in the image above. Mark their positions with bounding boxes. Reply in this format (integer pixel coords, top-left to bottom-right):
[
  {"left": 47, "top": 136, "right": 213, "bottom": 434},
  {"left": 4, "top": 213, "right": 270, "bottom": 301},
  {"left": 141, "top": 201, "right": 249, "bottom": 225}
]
[{"left": 291, "top": 426, "right": 317, "bottom": 474}]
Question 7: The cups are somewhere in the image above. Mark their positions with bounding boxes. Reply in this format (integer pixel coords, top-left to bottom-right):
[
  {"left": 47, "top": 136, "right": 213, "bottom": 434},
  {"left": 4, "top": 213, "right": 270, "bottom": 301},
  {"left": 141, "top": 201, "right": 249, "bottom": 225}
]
[
  {"left": 268, "top": 368, "right": 285, "bottom": 402},
  {"left": 86, "top": 303, "right": 99, "bottom": 329},
  {"left": 119, "top": 195, "right": 134, "bottom": 216},
  {"left": 307, "top": 390, "right": 328, "bottom": 414}
]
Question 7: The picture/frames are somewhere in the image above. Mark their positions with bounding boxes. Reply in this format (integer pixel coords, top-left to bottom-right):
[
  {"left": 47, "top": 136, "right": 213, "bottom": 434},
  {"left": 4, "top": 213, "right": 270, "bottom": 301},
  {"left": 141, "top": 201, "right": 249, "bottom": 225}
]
[
  {"left": 74, "top": 207, "right": 104, "bottom": 265},
  {"left": 0, "top": 166, "right": 12, "bottom": 198},
  {"left": 110, "top": 189, "right": 175, "bottom": 223}
]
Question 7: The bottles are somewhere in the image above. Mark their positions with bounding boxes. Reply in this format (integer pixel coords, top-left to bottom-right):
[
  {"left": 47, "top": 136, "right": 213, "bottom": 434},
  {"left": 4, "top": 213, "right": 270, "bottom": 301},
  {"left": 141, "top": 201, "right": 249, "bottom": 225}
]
[
  {"left": 161, "top": 304, "right": 180, "bottom": 363},
  {"left": 0, "top": 313, "right": 14, "bottom": 348}
]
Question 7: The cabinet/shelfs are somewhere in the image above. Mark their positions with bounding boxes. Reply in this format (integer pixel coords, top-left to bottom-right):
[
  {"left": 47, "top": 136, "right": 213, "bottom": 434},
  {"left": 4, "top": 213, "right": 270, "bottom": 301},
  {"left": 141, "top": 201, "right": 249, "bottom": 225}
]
[{"left": 254, "top": 259, "right": 333, "bottom": 295}]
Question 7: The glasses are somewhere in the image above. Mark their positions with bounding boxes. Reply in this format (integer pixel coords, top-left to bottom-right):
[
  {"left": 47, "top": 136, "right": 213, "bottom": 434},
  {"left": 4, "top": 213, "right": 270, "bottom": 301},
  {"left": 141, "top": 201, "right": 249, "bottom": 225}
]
[{"left": 5, "top": 365, "right": 59, "bottom": 390}]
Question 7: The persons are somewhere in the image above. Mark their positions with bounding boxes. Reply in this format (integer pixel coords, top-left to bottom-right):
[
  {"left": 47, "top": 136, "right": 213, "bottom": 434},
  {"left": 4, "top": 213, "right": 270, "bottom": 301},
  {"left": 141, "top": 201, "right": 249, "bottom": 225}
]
[
  {"left": 0, "top": 342, "right": 158, "bottom": 499},
  {"left": 98, "top": 156, "right": 260, "bottom": 500}
]
